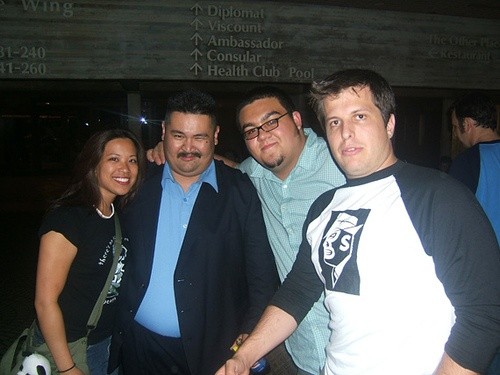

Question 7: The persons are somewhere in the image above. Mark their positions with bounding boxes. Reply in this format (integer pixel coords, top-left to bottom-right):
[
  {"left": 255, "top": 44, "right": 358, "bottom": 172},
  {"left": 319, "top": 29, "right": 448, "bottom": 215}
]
[
  {"left": 118, "top": 69, "right": 500, "bottom": 375},
  {"left": 26, "top": 130, "right": 139, "bottom": 375}
]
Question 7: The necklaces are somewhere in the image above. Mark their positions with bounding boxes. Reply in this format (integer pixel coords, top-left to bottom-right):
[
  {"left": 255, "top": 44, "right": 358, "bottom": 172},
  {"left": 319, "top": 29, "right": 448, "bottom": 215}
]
[{"left": 93, "top": 202, "right": 115, "bottom": 219}]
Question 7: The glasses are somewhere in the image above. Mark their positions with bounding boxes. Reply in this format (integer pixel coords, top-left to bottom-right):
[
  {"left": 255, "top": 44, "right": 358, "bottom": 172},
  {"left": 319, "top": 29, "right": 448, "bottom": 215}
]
[{"left": 242, "top": 112, "right": 288, "bottom": 141}]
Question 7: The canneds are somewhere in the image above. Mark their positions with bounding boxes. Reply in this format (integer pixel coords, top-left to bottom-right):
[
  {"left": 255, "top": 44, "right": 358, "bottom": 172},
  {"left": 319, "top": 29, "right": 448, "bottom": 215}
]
[{"left": 232, "top": 338, "right": 266, "bottom": 372}]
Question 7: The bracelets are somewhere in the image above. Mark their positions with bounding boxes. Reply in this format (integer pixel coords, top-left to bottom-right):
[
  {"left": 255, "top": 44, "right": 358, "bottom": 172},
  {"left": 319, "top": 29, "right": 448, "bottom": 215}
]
[{"left": 58, "top": 363, "right": 76, "bottom": 373}]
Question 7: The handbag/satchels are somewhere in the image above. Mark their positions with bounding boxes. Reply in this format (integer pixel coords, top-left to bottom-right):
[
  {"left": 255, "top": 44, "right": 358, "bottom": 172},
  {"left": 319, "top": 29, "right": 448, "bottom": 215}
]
[{"left": 0, "top": 324, "right": 90, "bottom": 375}]
